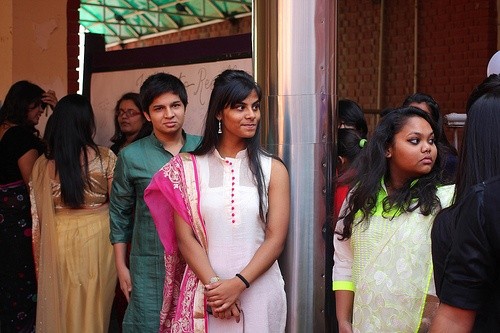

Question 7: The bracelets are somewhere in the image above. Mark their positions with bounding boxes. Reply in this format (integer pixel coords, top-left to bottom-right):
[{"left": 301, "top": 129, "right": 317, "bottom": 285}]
[
  {"left": 235, "top": 272, "right": 251, "bottom": 289},
  {"left": 209, "top": 274, "right": 219, "bottom": 283}
]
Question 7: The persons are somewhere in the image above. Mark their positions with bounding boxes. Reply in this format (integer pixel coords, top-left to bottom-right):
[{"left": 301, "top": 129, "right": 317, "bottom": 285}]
[
  {"left": 331, "top": 51, "right": 500, "bottom": 332},
  {"left": 104, "top": 90, "right": 155, "bottom": 333},
  {"left": 107, "top": 71, "right": 218, "bottom": 332},
  {"left": 30, "top": 94, "right": 119, "bottom": 332},
  {"left": 0, "top": 78, "right": 57, "bottom": 333},
  {"left": 171, "top": 69, "right": 291, "bottom": 333}
]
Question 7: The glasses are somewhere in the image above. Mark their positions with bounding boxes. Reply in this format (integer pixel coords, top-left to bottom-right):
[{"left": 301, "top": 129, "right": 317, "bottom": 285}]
[{"left": 115, "top": 109, "right": 141, "bottom": 117}]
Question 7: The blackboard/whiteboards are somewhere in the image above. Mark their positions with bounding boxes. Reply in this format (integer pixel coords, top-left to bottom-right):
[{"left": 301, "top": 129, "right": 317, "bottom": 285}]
[{"left": 83, "top": 33, "right": 254, "bottom": 153}]
